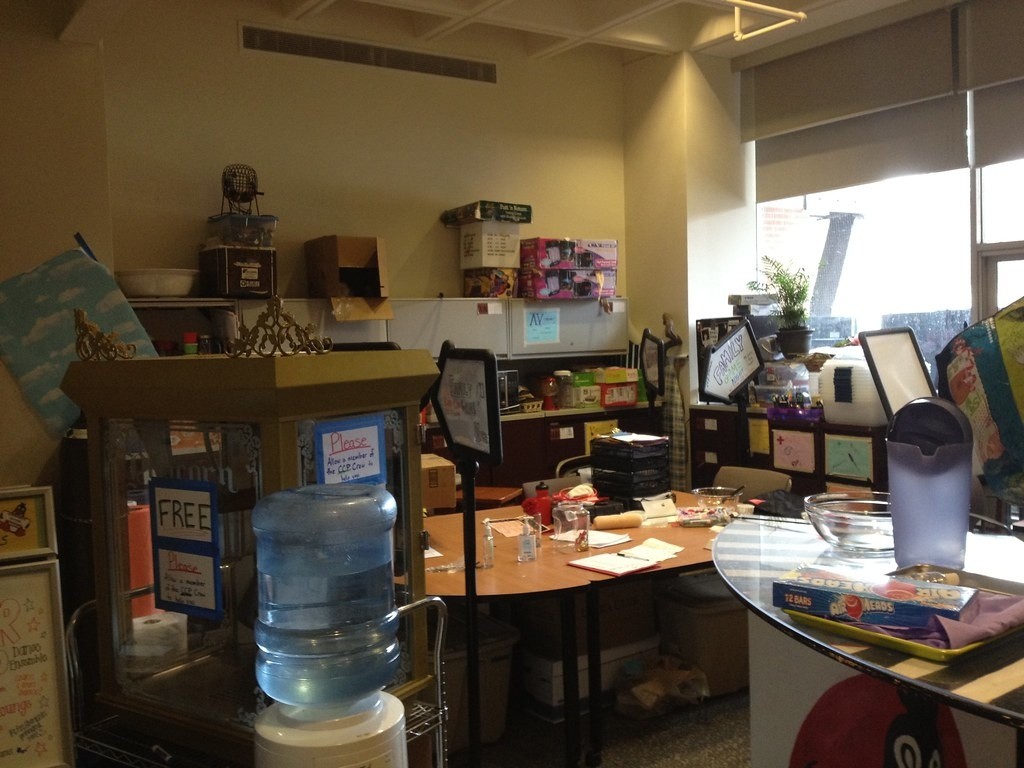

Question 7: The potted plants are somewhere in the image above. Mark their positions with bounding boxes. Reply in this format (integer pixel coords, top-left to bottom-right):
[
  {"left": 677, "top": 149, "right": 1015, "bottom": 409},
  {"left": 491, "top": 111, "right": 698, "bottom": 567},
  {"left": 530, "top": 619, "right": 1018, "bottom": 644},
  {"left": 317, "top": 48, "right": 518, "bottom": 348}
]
[{"left": 746, "top": 255, "right": 815, "bottom": 360}]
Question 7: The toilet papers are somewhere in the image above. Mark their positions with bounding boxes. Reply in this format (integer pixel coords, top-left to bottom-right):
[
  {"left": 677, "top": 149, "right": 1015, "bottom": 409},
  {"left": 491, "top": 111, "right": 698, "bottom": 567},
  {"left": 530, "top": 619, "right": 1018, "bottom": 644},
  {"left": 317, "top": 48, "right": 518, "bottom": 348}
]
[{"left": 132, "top": 611, "right": 188, "bottom": 672}]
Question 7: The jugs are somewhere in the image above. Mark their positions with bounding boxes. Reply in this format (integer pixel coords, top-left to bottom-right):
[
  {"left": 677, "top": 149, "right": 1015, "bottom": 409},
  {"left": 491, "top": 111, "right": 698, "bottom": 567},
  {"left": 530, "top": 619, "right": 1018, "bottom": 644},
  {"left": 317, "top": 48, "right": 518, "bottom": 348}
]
[{"left": 884, "top": 398, "right": 972, "bottom": 574}]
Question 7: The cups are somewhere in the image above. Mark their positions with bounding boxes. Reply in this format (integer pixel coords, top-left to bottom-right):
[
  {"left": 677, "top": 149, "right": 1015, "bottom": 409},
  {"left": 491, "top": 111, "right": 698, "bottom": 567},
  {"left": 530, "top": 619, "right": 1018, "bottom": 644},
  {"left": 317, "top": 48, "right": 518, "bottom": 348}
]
[
  {"left": 198, "top": 335, "right": 222, "bottom": 354},
  {"left": 183, "top": 332, "right": 198, "bottom": 354},
  {"left": 521, "top": 513, "right": 541, "bottom": 547}
]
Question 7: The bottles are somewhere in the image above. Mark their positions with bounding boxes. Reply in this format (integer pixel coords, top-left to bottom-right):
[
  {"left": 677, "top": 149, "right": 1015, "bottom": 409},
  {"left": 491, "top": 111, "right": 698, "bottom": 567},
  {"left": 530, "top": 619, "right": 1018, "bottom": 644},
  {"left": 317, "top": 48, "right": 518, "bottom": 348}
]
[
  {"left": 553, "top": 499, "right": 589, "bottom": 553},
  {"left": 543, "top": 377, "right": 559, "bottom": 411},
  {"left": 552, "top": 370, "right": 573, "bottom": 409},
  {"left": 251, "top": 482, "right": 401, "bottom": 710}
]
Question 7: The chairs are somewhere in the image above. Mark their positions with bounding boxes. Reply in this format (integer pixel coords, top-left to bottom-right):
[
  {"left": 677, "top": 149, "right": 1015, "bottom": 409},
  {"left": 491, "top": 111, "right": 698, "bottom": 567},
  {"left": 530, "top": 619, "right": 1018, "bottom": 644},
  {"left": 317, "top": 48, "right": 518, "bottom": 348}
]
[
  {"left": 522, "top": 475, "right": 581, "bottom": 499},
  {"left": 555, "top": 453, "right": 634, "bottom": 514},
  {"left": 710, "top": 465, "right": 792, "bottom": 505}
]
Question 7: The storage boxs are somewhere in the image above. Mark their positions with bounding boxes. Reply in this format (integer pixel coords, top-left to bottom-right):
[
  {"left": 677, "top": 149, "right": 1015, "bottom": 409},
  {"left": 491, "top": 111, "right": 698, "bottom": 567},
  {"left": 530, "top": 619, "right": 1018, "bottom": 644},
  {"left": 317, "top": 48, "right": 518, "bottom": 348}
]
[
  {"left": 421, "top": 453, "right": 458, "bottom": 508},
  {"left": 558, "top": 367, "right": 639, "bottom": 410},
  {"left": 304, "top": 234, "right": 396, "bottom": 323},
  {"left": 440, "top": 201, "right": 618, "bottom": 301},
  {"left": 396, "top": 563, "right": 751, "bottom": 760},
  {"left": 772, "top": 562, "right": 981, "bottom": 632},
  {"left": 198, "top": 215, "right": 279, "bottom": 300}
]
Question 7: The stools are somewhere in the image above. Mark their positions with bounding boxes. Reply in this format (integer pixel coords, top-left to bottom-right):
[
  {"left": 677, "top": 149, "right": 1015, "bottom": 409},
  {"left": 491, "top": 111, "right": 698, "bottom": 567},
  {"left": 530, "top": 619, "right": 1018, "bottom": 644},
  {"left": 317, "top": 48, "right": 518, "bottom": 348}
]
[{"left": 454, "top": 486, "right": 523, "bottom": 514}]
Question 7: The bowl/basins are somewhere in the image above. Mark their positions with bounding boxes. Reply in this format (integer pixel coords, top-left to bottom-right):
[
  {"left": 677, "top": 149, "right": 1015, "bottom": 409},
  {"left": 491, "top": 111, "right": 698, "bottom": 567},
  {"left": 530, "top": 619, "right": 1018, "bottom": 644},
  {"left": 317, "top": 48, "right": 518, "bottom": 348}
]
[
  {"left": 803, "top": 493, "right": 903, "bottom": 552},
  {"left": 113, "top": 268, "right": 203, "bottom": 297},
  {"left": 692, "top": 486, "right": 742, "bottom": 512}
]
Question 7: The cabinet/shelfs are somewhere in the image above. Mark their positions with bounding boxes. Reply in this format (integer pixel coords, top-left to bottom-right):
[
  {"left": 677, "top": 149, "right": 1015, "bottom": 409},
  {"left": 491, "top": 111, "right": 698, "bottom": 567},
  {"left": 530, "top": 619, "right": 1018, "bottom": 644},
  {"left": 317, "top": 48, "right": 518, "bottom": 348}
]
[
  {"left": 767, "top": 417, "right": 888, "bottom": 518},
  {"left": 240, "top": 297, "right": 631, "bottom": 363},
  {"left": 63, "top": 582, "right": 452, "bottom": 768}
]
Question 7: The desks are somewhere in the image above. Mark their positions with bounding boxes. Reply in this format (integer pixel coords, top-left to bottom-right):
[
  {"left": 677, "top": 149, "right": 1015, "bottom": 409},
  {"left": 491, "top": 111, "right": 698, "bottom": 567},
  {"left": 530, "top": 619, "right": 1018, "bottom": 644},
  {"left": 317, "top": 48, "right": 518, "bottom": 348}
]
[
  {"left": 395, "top": 490, "right": 754, "bottom": 768},
  {"left": 420, "top": 398, "right": 662, "bottom": 514},
  {"left": 710, "top": 490, "right": 1024, "bottom": 768},
  {"left": 690, "top": 397, "right": 768, "bottom": 494}
]
